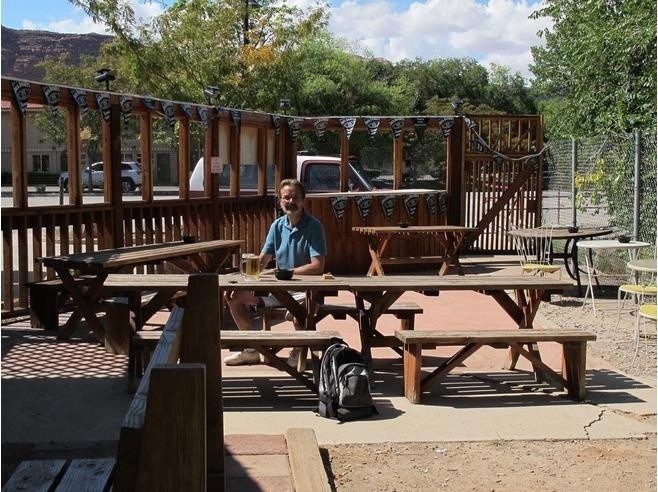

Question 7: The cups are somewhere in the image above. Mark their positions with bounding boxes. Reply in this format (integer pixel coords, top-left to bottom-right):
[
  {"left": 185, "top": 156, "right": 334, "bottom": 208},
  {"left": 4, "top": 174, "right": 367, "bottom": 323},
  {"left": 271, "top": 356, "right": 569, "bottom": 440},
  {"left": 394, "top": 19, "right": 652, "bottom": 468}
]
[{"left": 240, "top": 253, "right": 261, "bottom": 282}]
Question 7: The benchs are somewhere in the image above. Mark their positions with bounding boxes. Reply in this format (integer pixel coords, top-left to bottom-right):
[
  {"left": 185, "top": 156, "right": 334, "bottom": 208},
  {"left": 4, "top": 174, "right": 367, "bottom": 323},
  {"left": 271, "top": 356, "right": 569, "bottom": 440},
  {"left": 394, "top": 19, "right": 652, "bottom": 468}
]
[
  {"left": 129, "top": 330, "right": 343, "bottom": 404},
  {"left": 2, "top": 273, "right": 225, "bottom": 491},
  {"left": 394, "top": 328, "right": 597, "bottom": 406},
  {"left": 261, "top": 299, "right": 424, "bottom": 365}
]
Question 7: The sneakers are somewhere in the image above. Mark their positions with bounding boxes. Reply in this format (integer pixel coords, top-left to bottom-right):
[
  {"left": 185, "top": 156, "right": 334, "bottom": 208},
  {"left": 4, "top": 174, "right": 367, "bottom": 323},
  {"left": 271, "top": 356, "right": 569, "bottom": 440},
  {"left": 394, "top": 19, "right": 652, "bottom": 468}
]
[
  {"left": 285, "top": 347, "right": 301, "bottom": 369},
  {"left": 224, "top": 350, "right": 262, "bottom": 366}
]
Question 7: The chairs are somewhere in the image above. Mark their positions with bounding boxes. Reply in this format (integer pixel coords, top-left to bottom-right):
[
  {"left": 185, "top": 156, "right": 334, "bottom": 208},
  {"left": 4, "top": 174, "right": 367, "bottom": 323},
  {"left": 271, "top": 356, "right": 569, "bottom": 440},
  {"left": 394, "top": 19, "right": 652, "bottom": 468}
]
[
  {"left": 510, "top": 224, "right": 563, "bottom": 307},
  {"left": 613, "top": 258, "right": 657, "bottom": 373}
]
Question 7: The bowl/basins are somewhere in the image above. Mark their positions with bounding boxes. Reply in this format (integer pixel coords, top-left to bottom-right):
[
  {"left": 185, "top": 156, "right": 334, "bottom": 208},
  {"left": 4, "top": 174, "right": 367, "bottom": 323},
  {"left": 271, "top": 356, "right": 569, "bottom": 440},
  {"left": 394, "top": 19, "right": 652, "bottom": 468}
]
[
  {"left": 274, "top": 268, "right": 294, "bottom": 280},
  {"left": 398, "top": 222, "right": 409, "bottom": 228},
  {"left": 618, "top": 236, "right": 631, "bottom": 243},
  {"left": 568, "top": 227, "right": 579, "bottom": 233},
  {"left": 183, "top": 236, "right": 196, "bottom": 244}
]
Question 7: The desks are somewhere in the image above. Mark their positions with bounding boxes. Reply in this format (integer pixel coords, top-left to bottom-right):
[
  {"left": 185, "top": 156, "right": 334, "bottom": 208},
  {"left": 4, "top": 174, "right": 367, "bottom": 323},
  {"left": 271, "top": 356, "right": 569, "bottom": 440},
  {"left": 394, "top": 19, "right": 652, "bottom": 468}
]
[
  {"left": 351, "top": 225, "right": 480, "bottom": 277},
  {"left": 33, "top": 240, "right": 243, "bottom": 347},
  {"left": 347, "top": 276, "right": 572, "bottom": 388},
  {"left": 104, "top": 272, "right": 348, "bottom": 385},
  {"left": 575, "top": 239, "right": 650, "bottom": 315},
  {"left": 505, "top": 227, "right": 612, "bottom": 298}
]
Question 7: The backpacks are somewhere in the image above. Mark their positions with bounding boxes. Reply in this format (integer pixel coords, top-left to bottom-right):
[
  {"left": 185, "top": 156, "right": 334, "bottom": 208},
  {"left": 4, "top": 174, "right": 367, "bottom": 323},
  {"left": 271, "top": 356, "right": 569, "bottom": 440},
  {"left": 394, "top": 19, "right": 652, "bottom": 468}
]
[{"left": 317, "top": 337, "right": 378, "bottom": 423}]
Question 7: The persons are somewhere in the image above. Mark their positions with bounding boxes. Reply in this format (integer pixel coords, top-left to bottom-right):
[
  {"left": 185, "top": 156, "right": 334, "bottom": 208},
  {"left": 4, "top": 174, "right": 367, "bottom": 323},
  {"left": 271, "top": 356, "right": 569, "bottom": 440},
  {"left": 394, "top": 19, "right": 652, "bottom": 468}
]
[{"left": 221, "top": 178, "right": 329, "bottom": 368}]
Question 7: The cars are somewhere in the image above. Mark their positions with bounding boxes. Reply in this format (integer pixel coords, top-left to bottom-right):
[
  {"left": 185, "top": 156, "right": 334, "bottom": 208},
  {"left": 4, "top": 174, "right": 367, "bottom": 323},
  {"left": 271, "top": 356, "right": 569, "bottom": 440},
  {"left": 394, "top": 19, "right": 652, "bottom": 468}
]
[{"left": 58, "top": 162, "right": 142, "bottom": 193}]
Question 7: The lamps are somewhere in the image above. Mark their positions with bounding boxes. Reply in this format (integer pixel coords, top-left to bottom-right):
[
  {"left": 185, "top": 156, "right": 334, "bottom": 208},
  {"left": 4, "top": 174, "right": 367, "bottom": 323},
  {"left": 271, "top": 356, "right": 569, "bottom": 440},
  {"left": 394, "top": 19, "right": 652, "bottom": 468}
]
[
  {"left": 204, "top": 85, "right": 221, "bottom": 106},
  {"left": 95, "top": 68, "right": 115, "bottom": 91},
  {"left": 279, "top": 100, "right": 290, "bottom": 115},
  {"left": 450, "top": 100, "right": 464, "bottom": 114}
]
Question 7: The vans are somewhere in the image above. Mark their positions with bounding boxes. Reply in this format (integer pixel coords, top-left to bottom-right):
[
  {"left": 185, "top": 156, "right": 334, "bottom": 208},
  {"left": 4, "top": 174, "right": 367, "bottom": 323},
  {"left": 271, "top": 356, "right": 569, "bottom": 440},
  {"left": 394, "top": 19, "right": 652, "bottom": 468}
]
[{"left": 189, "top": 154, "right": 376, "bottom": 199}]
